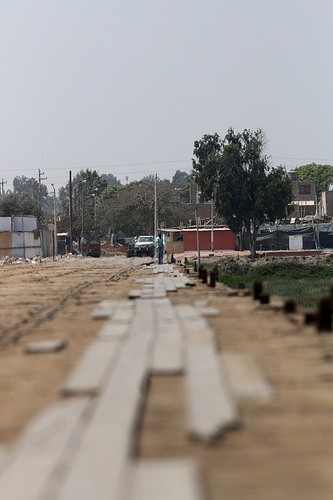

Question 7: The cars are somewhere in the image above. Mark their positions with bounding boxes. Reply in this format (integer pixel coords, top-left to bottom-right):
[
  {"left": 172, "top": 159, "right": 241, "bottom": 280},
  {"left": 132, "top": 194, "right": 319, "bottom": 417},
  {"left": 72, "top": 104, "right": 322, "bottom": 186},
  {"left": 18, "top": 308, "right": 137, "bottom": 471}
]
[{"left": 126, "top": 235, "right": 154, "bottom": 256}]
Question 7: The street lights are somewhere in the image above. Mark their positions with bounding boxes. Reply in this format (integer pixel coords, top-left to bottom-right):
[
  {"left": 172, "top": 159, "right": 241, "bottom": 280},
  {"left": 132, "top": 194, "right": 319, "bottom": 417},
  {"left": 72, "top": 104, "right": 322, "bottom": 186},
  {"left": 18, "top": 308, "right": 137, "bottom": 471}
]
[{"left": 50, "top": 182, "right": 58, "bottom": 256}]
